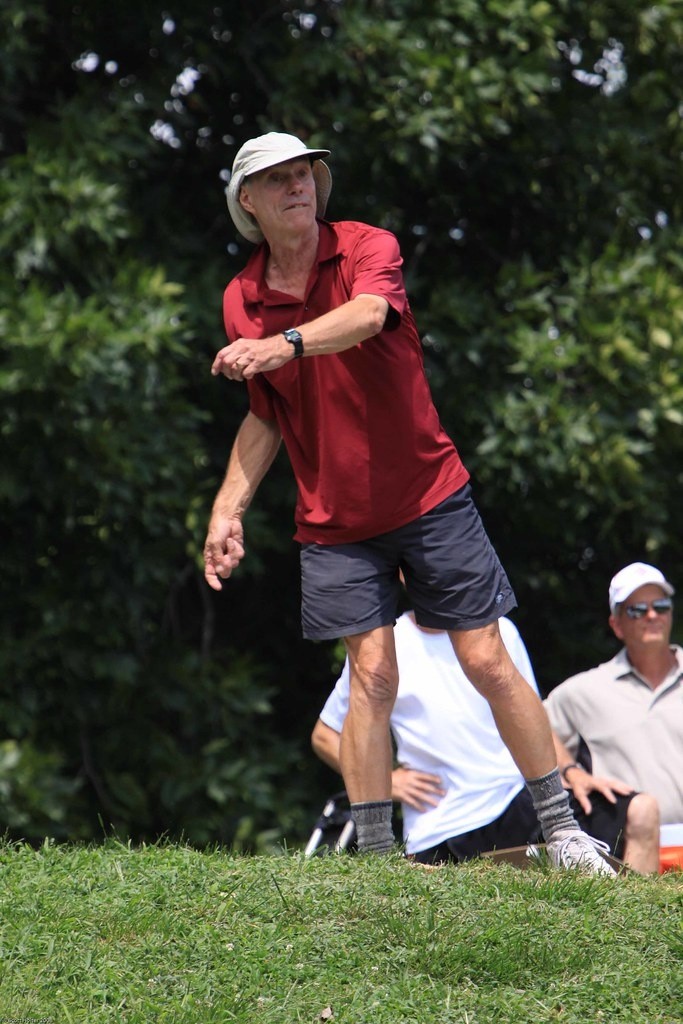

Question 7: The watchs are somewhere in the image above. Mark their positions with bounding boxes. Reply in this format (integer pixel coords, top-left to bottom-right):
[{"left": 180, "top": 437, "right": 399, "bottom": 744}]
[{"left": 282, "top": 328, "right": 304, "bottom": 359}]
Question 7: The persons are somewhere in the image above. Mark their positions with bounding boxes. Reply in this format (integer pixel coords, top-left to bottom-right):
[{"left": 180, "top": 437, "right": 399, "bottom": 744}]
[
  {"left": 543, "top": 562, "right": 683, "bottom": 824},
  {"left": 311, "top": 566, "right": 661, "bottom": 881},
  {"left": 203, "top": 132, "right": 617, "bottom": 882}
]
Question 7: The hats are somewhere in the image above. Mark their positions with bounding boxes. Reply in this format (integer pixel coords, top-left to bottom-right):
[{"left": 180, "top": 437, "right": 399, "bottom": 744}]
[
  {"left": 609, "top": 561, "right": 678, "bottom": 611},
  {"left": 223, "top": 130, "right": 332, "bottom": 244}
]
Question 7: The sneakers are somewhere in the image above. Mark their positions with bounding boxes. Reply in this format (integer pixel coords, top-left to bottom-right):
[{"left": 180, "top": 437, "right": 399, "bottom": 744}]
[{"left": 543, "top": 826, "right": 620, "bottom": 883}]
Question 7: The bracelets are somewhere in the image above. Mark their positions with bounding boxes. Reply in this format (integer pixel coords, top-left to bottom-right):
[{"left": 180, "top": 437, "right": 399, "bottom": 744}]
[{"left": 563, "top": 763, "right": 580, "bottom": 784}]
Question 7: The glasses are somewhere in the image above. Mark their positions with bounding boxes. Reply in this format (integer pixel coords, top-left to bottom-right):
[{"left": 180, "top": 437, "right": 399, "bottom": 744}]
[{"left": 617, "top": 596, "right": 674, "bottom": 620}]
